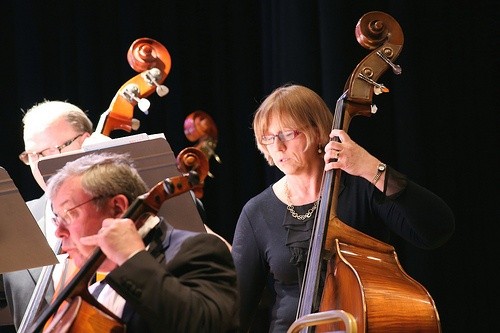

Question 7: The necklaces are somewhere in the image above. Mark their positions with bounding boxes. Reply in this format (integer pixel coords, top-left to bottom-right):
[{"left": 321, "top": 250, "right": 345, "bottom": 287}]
[{"left": 284, "top": 176, "right": 326, "bottom": 221}]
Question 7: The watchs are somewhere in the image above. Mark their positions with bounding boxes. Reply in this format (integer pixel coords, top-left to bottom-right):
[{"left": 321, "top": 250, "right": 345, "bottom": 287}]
[{"left": 371, "top": 163, "right": 386, "bottom": 186}]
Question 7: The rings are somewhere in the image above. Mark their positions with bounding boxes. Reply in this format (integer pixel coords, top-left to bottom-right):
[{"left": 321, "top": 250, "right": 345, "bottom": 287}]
[{"left": 336, "top": 150, "right": 339, "bottom": 158}]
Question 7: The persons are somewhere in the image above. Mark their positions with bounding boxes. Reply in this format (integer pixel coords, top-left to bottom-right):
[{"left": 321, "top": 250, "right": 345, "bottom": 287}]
[
  {"left": 231, "top": 85, "right": 456, "bottom": 333},
  {"left": 43, "top": 153, "right": 239, "bottom": 333},
  {"left": 0, "top": 100, "right": 232, "bottom": 333}
]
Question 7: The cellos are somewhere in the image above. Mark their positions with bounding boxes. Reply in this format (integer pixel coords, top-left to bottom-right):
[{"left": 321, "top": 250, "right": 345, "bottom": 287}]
[
  {"left": 24, "top": 110, "right": 218, "bottom": 333},
  {"left": 16, "top": 37, "right": 175, "bottom": 333},
  {"left": 287, "top": 11, "right": 443, "bottom": 333}
]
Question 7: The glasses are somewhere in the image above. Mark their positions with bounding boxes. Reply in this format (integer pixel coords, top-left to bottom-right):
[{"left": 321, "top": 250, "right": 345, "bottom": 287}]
[
  {"left": 19, "top": 134, "right": 83, "bottom": 166},
  {"left": 260, "top": 124, "right": 308, "bottom": 145},
  {"left": 50, "top": 195, "right": 103, "bottom": 227}
]
